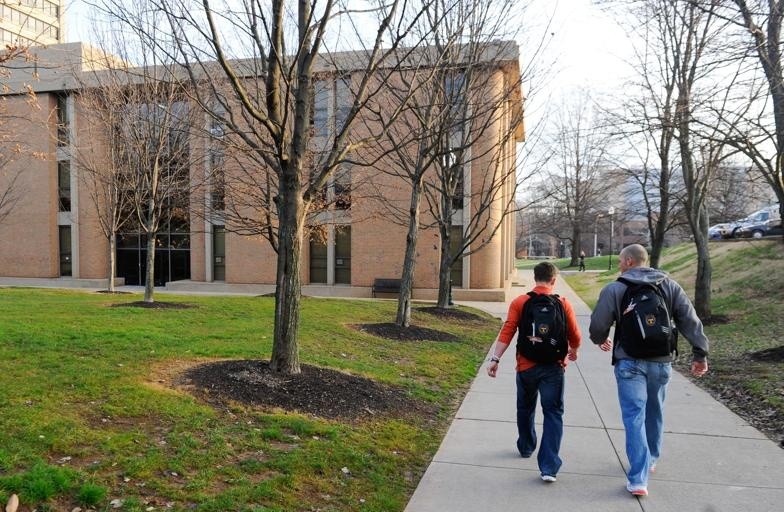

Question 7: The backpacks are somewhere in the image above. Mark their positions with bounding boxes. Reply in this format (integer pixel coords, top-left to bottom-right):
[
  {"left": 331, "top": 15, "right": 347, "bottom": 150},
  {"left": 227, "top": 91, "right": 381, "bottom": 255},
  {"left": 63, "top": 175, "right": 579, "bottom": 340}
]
[
  {"left": 517, "top": 292, "right": 567, "bottom": 363},
  {"left": 616, "top": 277, "right": 678, "bottom": 359}
]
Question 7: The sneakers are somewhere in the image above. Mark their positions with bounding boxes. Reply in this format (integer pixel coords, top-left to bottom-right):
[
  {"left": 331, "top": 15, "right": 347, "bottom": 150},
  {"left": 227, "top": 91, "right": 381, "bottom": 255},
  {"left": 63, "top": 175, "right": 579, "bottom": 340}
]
[
  {"left": 627, "top": 465, "right": 655, "bottom": 495},
  {"left": 542, "top": 475, "right": 556, "bottom": 482}
]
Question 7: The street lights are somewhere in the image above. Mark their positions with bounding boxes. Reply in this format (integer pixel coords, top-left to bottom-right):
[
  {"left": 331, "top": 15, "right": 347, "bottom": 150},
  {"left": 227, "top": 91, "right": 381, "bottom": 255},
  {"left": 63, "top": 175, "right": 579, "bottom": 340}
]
[
  {"left": 443, "top": 148, "right": 454, "bottom": 306},
  {"left": 609, "top": 207, "right": 614, "bottom": 269}
]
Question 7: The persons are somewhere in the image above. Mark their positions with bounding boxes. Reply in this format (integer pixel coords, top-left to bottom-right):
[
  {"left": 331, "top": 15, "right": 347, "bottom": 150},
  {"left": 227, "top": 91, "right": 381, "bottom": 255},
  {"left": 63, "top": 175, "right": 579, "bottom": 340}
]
[
  {"left": 589, "top": 244, "right": 710, "bottom": 497},
  {"left": 578, "top": 251, "right": 586, "bottom": 272},
  {"left": 486, "top": 263, "right": 582, "bottom": 482}
]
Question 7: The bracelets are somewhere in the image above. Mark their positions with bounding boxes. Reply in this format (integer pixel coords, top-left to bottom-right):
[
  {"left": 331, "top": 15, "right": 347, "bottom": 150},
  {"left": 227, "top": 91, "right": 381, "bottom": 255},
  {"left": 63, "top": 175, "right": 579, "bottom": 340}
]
[{"left": 491, "top": 353, "right": 499, "bottom": 362}]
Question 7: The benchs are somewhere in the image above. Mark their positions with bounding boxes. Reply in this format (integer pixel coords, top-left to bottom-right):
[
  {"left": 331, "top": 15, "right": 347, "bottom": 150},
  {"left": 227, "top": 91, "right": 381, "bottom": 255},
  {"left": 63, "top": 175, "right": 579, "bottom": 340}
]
[{"left": 372, "top": 279, "right": 412, "bottom": 300}]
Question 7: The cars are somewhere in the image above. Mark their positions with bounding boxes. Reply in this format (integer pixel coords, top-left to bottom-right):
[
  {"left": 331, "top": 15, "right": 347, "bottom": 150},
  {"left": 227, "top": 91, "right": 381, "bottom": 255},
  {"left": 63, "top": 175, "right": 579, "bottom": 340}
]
[{"left": 709, "top": 205, "right": 781, "bottom": 238}]
